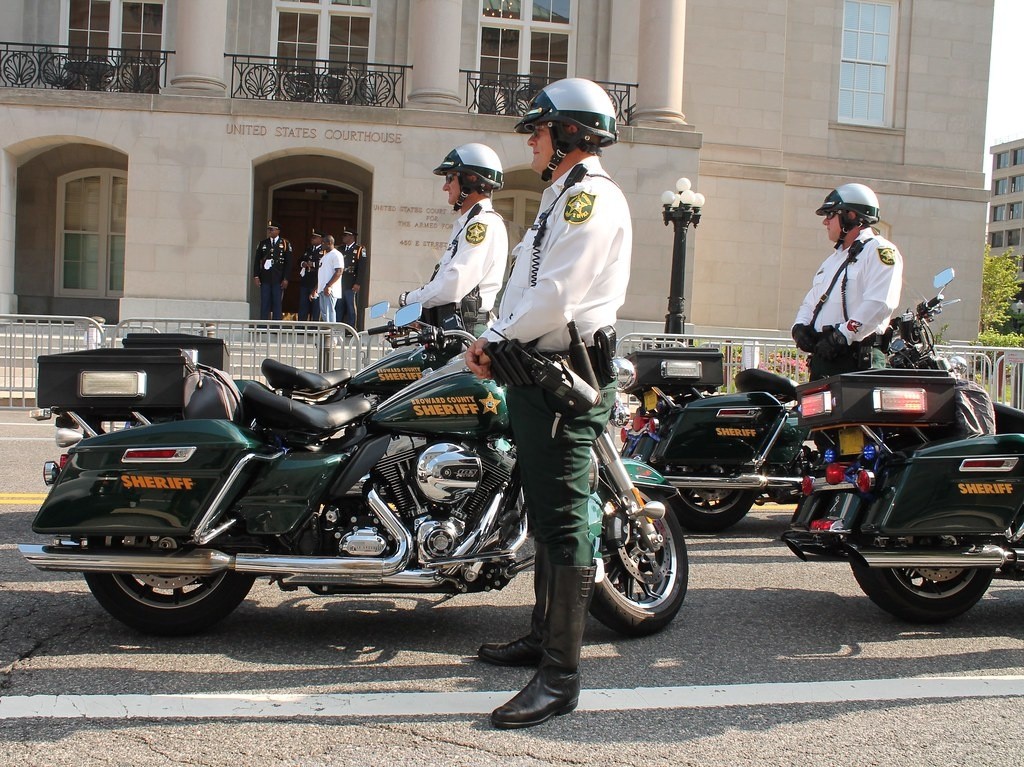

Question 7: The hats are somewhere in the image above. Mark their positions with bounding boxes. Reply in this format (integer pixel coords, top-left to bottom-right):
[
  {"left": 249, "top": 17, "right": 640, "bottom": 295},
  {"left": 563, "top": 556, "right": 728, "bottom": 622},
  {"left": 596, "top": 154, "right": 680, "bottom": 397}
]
[
  {"left": 267, "top": 219, "right": 281, "bottom": 230},
  {"left": 344, "top": 226, "right": 358, "bottom": 235},
  {"left": 312, "top": 228, "right": 325, "bottom": 237}
]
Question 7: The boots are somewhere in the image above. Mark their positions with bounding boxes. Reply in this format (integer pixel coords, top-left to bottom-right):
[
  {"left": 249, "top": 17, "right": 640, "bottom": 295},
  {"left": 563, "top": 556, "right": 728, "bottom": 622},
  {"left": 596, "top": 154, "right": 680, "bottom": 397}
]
[
  {"left": 493, "top": 559, "right": 597, "bottom": 727},
  {"left": 478, "top": 539, "right": 560, "bottom": 666}
]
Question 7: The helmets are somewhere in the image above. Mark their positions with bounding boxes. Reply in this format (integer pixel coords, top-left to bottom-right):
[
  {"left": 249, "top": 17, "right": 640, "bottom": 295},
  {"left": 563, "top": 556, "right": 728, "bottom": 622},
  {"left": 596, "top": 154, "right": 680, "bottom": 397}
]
[
  {"left": 433, "top": 143, "right": 504, "bottom": 189},
  {"left": 514, "top": 78, "right": 619, "bottom": 141},
  {"left": 815, "top": 183, "right": 881, "bottom": 224}
]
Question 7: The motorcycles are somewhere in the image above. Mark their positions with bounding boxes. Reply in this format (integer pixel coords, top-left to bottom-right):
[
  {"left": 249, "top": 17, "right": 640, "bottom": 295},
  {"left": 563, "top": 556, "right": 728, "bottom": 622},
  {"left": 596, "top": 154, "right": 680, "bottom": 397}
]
[{"left": 16, "top": 266, "right": 1024, "bottom": 637}]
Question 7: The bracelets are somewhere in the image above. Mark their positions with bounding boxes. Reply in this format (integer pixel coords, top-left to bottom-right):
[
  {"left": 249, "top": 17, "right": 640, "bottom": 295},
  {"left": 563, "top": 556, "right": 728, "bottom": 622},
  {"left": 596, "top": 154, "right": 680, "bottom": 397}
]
[
  {"left": 326, "top": 284, "right": 330, "bottom": 286},
  {"left": 401, "top": 291, "right": 409, "bottom": 306},
  {"left": 255, "top": 276, "right": 258, "bottom": 278}
]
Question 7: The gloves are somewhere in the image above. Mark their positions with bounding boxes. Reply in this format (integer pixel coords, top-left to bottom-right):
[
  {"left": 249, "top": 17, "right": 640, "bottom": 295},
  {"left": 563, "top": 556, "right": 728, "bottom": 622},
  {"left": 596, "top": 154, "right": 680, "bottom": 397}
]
[
  {"left": 300, "top": 268, "right": 306, "bottom": 277},
  {"left": 792, "top": 324, "right": 818, "bottom": 352},
  {"left": 814, "top": 329, "right": 848, "bottom": 361},
  {"left": 264, "top": 259, "right": 273, "bottom": 269},
  {"left": 399, "top": 291, "right": 410, "bottom": 307}
]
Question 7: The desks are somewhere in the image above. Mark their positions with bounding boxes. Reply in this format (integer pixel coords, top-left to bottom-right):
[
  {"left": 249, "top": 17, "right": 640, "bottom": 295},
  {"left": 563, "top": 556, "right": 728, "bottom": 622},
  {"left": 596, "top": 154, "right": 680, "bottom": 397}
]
[
  {"left": 67, "top": 59, "right": 111, "bottom": 90},
  {"left": 295, "top": 73, "right": 343, "bottom": 102}
]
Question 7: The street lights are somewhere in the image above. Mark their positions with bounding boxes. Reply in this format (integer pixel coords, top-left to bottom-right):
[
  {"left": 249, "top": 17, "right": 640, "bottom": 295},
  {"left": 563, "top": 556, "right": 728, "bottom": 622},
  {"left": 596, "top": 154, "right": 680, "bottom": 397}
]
[{"left": 660, "top": 176, "right": 707, "bottom": 349}]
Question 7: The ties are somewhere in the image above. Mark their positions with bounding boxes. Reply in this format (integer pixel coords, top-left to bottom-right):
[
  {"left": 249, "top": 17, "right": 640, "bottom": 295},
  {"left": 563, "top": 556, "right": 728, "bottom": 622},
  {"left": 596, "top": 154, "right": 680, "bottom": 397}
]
[
  {"left": 273, "top": 239, "right": 275, "bottom": 247},
  {"left": 347, "top": 246, "right": 349, "bottom": 250},
  {"left": 313, "top": 246, "right": 316, "bottom": 251}
]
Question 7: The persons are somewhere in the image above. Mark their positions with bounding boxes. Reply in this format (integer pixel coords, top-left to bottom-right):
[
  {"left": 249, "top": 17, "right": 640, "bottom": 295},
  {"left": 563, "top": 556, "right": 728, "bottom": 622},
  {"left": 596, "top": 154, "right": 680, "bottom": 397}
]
[
  {"left": 296, "top": 227, "right": 325, "bottom": 335},
  {"left": 336, "top": 224, "right": 367, "bottom": 335},
  {"left": 310, "top": 234, "right": 345, "bottom": 346},
  {"left": 253, "top": 219, "right": 294, "bottom": 333},
  {"left": 464, "top": 78, "right": 635, "bottom": 731},
  {"left": 397, "top": 144, "right": 509, "bottom": 340},
  {"left": 792, "top": 184, "right": 903, "bottom": 386}
]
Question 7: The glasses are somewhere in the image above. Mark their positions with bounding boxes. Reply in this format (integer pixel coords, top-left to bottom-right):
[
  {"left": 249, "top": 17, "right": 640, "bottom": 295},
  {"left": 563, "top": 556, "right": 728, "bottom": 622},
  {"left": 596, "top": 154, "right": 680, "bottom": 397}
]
[
  {"left": 446, "top": 173, "right": 458, "bottom": 183},
  {"left": 827, "top": 211, "right": 839, "bottom": 219}
]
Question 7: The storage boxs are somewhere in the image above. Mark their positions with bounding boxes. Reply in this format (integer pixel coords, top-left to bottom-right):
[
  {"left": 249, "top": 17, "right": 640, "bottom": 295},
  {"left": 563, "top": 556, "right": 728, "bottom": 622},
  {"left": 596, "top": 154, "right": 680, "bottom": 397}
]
[
  {"left": 653, "top": 392, "right": 787, "bottom": 465},
  {"left": 35, "top": 347, "right": 199, "bottom": 406},
  {"left": 25, "top": 420, "right": 281, "bottom": 538},
  {"left": 794, "top": 367, "right": 955, "bottom": 427},
  {"left": 859, "top": 433, "right": 1024, "bottom": 536},
  {"left": 623, "top": 346, "right": 724, "bottom": 391},
  {"left": 122, "top": 332, "right": 230, "bottom": 375}
]
